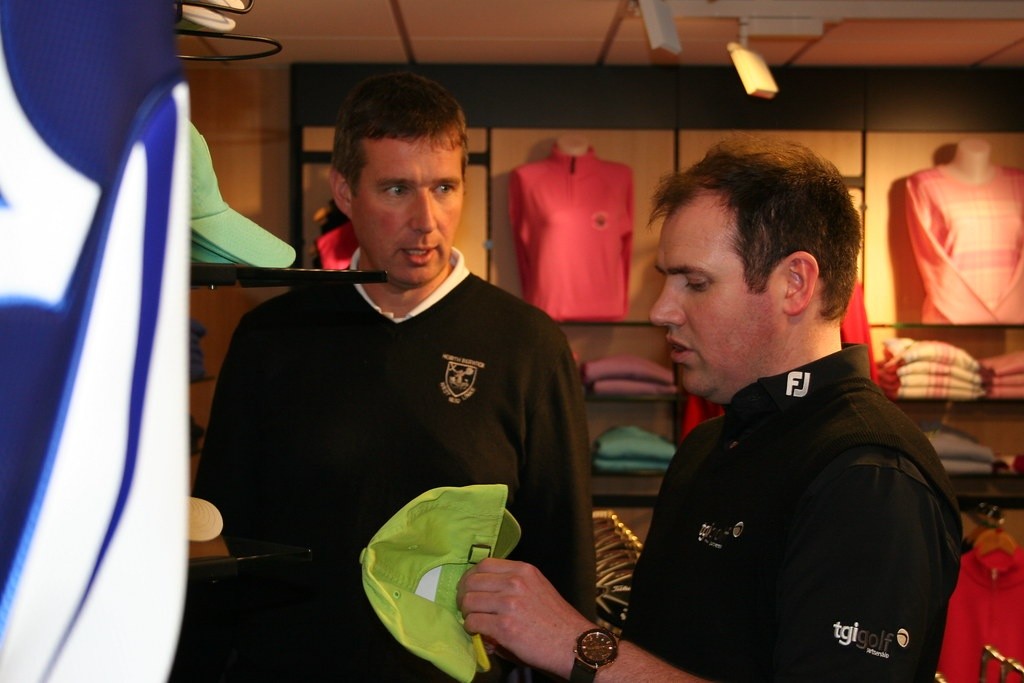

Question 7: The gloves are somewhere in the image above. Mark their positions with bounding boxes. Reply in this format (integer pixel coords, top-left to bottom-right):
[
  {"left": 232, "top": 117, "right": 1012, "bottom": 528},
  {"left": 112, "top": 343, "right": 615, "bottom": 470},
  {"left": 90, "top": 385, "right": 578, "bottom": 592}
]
[{"left": 181, "top": 0, "right": 244, "bottom": 32}]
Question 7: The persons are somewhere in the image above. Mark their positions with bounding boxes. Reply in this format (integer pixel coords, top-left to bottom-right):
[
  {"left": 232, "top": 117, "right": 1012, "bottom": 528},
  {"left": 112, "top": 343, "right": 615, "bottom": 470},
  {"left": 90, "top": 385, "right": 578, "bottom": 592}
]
[
  {"left": 507, "top": 136, "right": 636, "bottom": 320},
  {"left": 449, "top": 142, "right": 963, "bottom": 683},
  {"left": 196, "top": 72, "right": 598, "bottom": 683},
  {"left": 905, "top": 139, "right": 1024, "bottom": 323}
]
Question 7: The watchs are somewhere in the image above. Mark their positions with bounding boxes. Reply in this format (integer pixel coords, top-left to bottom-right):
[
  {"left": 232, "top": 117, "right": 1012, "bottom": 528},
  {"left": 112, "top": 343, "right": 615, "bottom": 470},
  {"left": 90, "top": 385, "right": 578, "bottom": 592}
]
[{"left": 568, "top": 627, "right": 620, "bottom": 683}]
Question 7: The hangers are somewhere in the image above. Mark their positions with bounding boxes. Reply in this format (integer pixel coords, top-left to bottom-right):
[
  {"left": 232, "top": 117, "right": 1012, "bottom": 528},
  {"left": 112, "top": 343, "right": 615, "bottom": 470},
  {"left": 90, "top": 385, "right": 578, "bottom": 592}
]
[{"left": 961, "top": 503, "right": 1024, "bottom": 575}]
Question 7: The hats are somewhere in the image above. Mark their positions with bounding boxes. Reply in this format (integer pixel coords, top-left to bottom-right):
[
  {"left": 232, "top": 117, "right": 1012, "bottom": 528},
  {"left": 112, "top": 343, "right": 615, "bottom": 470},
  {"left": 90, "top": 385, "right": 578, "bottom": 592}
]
[
  {"left": 188, "top": 120, "right": 298, "bottom": 269},
  {"left": 360, "top": 483, "right": 524, "bottom": 683}
]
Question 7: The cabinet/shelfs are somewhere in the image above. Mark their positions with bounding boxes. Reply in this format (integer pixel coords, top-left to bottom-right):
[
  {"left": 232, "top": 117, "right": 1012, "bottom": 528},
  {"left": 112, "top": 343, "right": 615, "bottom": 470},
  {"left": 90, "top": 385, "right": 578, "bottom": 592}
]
[
  {"left": 0, "top": 0, "right": 387, "bottom": 683},
  {"left": 290, "top": 60, "right": 1024, "bottom": 509}
]
